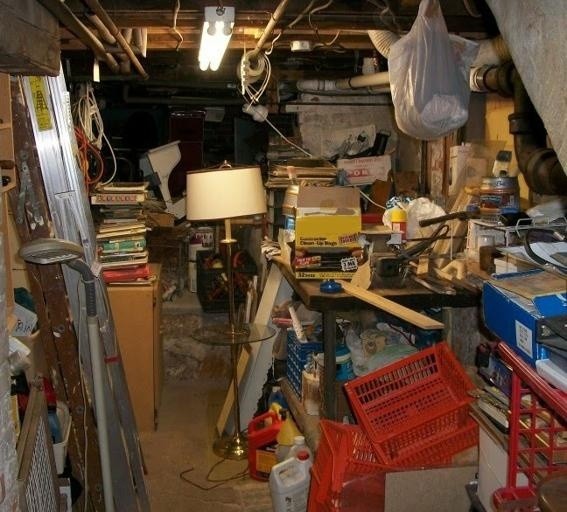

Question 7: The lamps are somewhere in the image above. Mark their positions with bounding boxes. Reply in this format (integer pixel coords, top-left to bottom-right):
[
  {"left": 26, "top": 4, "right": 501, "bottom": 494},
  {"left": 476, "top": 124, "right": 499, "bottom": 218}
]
[
  {"left": 184, "top": 160, "right": 276, "bottom": 461},
  {"left": 197, "top": 6, "right": 236, "bottom": 73}
]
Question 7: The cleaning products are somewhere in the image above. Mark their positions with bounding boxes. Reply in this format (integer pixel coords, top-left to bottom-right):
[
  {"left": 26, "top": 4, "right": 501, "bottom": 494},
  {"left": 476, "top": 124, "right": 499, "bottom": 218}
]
[{"left": 245, "top": 403, "right": 315, "bottom": 512}]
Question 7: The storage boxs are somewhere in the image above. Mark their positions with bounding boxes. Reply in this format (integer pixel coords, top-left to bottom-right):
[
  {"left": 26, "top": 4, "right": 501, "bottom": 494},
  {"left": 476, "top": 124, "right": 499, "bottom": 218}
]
[
  {"left": 50, "top": 414, "right": 72, "bottom": 476},
  {"left": 483, "top": 266, "right": 566, "bottom": 370},
  {"left": 312, "top": 420, "right": 395, "bottom": 506},
  {"left": 107, "top": 259, "right": 163, "bottom": 437},
  {"left": 195, "top": 250, "right": 257, "bottom": 313},
  {"left": 280, "top": 184, "right": 360, "bottom": 216},
  {"left": 336, "top": 154, "right": 392, "bottom": 188},
  {"left": 287, "top": 242, "right": 365, "bottom": 279},
  {"left": 283, "top": 325, "right": 345, "bottom": 403},
  {"left": 353, "top": 408, "right": 480, "bottom": 471},
  {"left": 295, "top": 206, "right": 362, "bottom": 246},
  {"left": 345, "top": 340, "right": 481, "bottom": 457},
  {"left": 305, "top": 465, "right": 384, "bottom": 512}
]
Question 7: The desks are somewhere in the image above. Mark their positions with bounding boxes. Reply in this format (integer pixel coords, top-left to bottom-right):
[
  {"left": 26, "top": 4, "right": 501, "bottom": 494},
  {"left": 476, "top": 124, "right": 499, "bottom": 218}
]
[
  {"left": 491, "top": 339, "right": 567, "bottom": 512},
  {"left": 272, "top": 259, "right": 483, "bottom": 462}
]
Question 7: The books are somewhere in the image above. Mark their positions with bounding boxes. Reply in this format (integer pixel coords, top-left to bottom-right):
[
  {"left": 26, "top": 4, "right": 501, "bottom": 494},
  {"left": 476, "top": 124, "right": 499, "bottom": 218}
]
[
  {"left": 91, "top": 184, "right": 162, "bottom": 284},
  {"left": 465, "top": 380, "right": 567, "bottom": 466}
]
